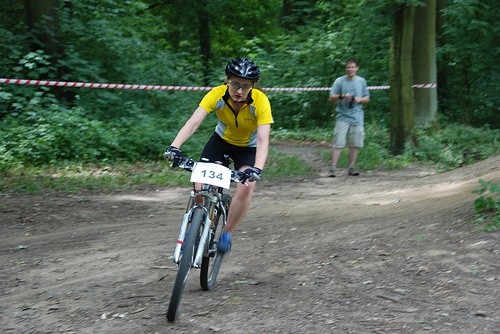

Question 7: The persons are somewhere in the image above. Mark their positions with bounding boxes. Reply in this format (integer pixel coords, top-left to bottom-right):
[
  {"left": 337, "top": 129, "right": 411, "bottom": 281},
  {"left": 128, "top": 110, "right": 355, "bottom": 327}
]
[
  {"left": 164, "top": 56, "right": 274, "bottom": 255},
  {"left": 328, "top": 59, "right": 370, "bottom": 178}
]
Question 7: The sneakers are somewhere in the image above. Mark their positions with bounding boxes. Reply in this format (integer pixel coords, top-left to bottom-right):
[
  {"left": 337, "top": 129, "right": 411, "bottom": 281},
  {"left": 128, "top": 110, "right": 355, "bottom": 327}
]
[
  {"left": 348, "top": 167, "right": 359, "bottom": 176},
  {"left": 328, "top": 166, "right": 337, "bottom": 177},
  {"left": 217, "top": 231, "right": 233, "bottom": 252}
]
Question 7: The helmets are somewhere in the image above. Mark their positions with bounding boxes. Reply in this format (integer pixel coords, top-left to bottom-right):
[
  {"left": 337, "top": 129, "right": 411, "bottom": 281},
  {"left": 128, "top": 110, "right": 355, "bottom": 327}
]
[{"left": 225, "top": 57, "right": 260, "bottom": 82}]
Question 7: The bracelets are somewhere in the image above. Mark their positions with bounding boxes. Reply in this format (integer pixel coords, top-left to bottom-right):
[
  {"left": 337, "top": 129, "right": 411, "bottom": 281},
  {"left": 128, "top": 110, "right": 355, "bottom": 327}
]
[{"left": 339, "top": 94, "right": 344, "bottom": 99}]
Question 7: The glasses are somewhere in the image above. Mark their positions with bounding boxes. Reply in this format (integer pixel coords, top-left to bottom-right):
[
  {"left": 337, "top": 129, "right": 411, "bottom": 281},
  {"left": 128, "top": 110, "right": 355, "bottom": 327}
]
[{"left": 227, "top": 78, "right": 253, "bottom": 90}]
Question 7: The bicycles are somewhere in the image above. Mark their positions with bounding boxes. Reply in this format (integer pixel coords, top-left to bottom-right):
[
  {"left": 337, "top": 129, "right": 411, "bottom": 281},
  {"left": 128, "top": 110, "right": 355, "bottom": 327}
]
[{"left": 162, "top": 150, "right": 261, "bottom": 322}]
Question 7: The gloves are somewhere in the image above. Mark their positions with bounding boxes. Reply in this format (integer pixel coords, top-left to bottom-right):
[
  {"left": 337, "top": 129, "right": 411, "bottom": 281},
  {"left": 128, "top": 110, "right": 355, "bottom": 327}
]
[
  {"left": 163, "top": 145, "right": 183, "bottom": 167},
  {"left": 239, "top": 168, "right": 263, "bottom": 184}
]
